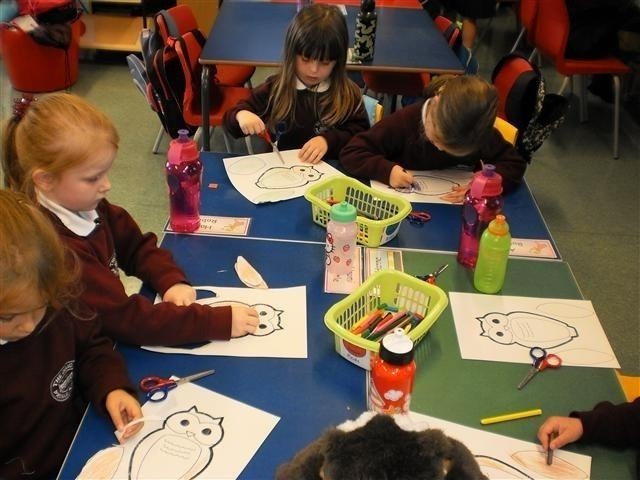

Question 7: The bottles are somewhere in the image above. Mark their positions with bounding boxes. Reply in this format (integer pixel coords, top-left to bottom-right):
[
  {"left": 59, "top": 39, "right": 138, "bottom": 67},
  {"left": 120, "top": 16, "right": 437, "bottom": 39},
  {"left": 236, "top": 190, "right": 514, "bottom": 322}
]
[
  {"left": 366, "top": 327, "right": 416, "bottom": 417},
  {"left": 351, "top": 0, "right": 377, "bottom": 64},
  {"left": 457, "top": 165, "right": 505, "bottom": 269},
  {"left": 324, "top": 201, "right": 359, "bottom": 275},
  {"left": 164, "top": 129, "right": 204, "bottom": 233},
  {"left": 473, "top": 214, "right": 512, "bottom": 295}
]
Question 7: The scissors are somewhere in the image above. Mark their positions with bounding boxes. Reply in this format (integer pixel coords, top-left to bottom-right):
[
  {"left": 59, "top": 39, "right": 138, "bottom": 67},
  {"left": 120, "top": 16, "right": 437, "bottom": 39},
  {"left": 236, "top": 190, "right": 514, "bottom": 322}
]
[
  {"left": 415, "top": 263, "right": 448, "bottom": 285},
  {"left": 140, "top": 369, "right": 216, "bottom": 402},
  {"left": 258, "top": 127, "right": 286, "bottom": 165},
  {"left": 517, "top": 347, "right": 562, "bottom": 390},
  {"left": 382, "top": 206, "right": 432, "bottom": 225}
]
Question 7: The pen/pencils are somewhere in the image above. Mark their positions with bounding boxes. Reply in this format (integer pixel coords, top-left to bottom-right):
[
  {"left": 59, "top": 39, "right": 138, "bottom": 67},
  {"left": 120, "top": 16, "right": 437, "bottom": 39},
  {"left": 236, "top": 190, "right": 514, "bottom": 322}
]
[
  {"left": 548, "top": 432, "right": 555, "bottom": 465},
  {"left": 348, "top": 303, "right": 425, "bottom": 343},
  {"left": 402, "top": 169, "right": 416, "bottom": 191},
  {"left": 480, "top": 409, "right": 542, "bottom": 425}
]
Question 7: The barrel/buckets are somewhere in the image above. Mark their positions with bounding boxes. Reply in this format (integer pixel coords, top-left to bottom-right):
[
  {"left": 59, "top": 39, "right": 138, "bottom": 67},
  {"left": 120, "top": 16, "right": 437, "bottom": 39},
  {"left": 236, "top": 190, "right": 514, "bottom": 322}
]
[{"left": 0, "top": 17, "right": 82, "bottom": 93}]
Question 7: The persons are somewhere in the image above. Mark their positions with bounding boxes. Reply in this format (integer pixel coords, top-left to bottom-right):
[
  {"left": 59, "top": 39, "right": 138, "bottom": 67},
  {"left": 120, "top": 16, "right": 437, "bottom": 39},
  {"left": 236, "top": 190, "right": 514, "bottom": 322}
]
[
  {"left": 1, "top": 189, "right": 147, "bottom": 479},
  {"left": 1, "top": 89, "right": 262, "bottom": 345},
  {"left": 536, "top": 393, "right": 640, "bottom": 452},
  {"left": 563, "top": 0, "right": 639, "bottom": 102},
  {"left": 221, "top": 1, "right": 371, "bottom": 165},
  {"left": 436, "top": 0, "right": 498, "bottom": 53},
  {"left": 336, "top": 73, "right": 527, "bottom": 195}
]
